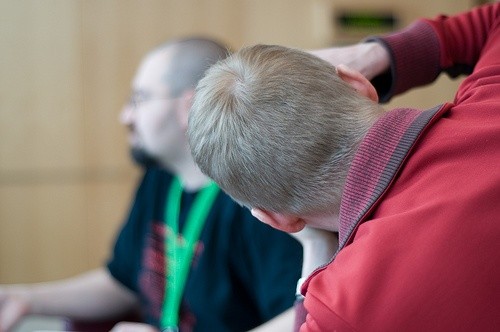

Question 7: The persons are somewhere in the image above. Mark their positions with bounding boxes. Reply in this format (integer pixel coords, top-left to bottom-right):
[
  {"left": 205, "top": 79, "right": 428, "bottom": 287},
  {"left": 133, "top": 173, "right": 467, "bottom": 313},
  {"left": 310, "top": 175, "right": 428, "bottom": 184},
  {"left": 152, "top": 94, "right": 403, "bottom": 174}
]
[
  {"left": 0, "top": 38, "right": 304, "bottom": 332},
  {"left": 188, "top": 1, "right": 500, "bottom": 332}
]
